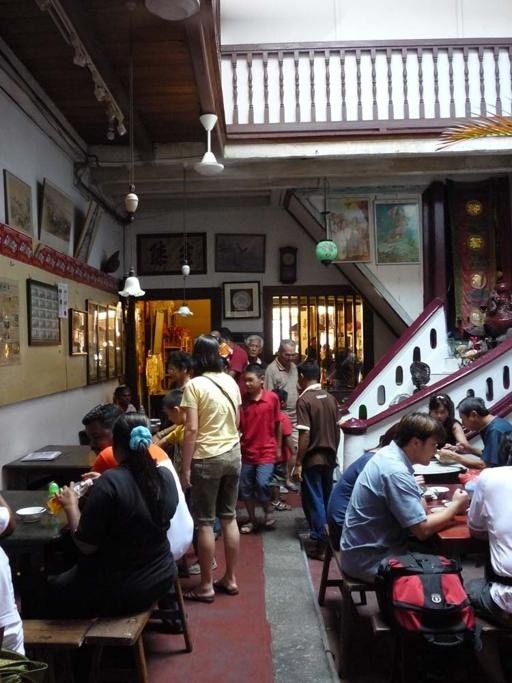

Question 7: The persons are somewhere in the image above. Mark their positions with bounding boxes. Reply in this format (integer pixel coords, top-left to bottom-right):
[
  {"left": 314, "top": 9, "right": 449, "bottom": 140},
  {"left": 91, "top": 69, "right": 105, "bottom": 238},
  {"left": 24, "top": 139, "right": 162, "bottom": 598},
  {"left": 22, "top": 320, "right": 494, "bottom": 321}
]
[
  {"left": 329, "top": 211, "right": 369, "bottom": 262},
  {"left": 1, "top": 494, "right": 28, "bottom": 679},
  {"left": 49, "top": 324, "right": 362, "bottom": 682},
  {"left": 326, "top": 392, "right": 511, "bottom": 629}
]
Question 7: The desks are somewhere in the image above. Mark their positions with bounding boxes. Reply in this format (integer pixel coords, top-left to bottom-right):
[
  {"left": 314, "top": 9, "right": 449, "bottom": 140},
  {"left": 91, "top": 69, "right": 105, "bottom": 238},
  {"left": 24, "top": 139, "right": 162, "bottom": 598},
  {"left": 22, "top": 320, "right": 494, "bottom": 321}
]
[
  {"left": 303, "top": 449, "right": 510, "bottom": 682},
  {"left": 0, "top": 409, "right": 212, "bottom": 681}
]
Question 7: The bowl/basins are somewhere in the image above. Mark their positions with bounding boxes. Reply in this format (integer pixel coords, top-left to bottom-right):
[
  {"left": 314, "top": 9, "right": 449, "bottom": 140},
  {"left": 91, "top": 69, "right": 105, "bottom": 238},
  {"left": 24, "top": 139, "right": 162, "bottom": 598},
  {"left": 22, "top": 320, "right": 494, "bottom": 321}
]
[
  {"left": 16, "top": 506, "right": 45, "bottom": 522},
  {"left": 424, "top": 485, "right": 466, "bottom": 515}
]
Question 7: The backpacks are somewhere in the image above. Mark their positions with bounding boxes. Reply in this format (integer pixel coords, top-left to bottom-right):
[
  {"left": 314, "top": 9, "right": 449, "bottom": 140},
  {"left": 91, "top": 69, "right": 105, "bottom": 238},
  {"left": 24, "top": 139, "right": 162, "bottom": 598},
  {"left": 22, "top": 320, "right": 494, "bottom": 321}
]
[{"left": 374, "top": 551, "right": 485, "bottom": 652}]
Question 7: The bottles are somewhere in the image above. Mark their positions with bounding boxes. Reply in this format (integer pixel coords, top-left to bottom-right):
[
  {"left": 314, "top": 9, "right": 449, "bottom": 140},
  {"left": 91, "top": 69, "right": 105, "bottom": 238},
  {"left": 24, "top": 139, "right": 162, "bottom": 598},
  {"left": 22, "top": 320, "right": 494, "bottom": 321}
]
[
  {"left": 138, "top": 404, "right": 145, "bottom": 414},
  {"left": 48, "top": 478, "right": 93, "bottom": 513}
]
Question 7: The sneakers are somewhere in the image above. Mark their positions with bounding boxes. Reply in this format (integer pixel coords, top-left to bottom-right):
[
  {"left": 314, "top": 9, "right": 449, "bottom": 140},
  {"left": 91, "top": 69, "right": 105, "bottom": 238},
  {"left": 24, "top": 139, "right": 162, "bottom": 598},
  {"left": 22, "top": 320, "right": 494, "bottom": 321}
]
[
  {"left": 186, "top": 555, "right": 217, "bottom": 574},
  {"left": 264, "top": 481, "right": 298, "bottom": 527}
]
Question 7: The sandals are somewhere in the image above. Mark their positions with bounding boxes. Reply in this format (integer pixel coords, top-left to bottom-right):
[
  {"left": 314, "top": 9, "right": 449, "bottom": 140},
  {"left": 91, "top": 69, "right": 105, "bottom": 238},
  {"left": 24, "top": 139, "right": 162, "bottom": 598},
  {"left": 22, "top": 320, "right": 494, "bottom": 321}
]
[{"left": 240, "top": 519, "right": 257, "bottom": 534}]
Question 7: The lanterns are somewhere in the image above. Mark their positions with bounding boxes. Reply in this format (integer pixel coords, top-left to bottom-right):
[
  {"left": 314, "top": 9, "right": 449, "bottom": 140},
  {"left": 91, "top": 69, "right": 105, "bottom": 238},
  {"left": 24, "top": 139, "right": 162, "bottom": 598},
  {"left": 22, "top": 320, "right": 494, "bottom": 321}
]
[{"left": 315, "top": 239, "right": 337, "bottom": 265}]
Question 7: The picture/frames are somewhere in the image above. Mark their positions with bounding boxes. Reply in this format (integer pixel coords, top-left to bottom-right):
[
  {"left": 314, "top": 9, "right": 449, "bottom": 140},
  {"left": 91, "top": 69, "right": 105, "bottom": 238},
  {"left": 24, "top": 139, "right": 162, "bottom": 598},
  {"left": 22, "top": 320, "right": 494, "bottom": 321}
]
[
  {"left": 38, "top": 173, "right": 77, "bottom": 258},
  {"left": 67, "top": 305, "right": 89, "bottom": 357},
  {"left": 133, "top": 230, "right": 209, "bottom": 277},
  {"left": 320, "top": 193, "right": 374, "bottom": 264},
  {"left": 212, "top": 231, "right": 266, "bottom": 274},
  {"left": 3, "top": 167, "right": 35, "bottom": 239},
  {"left": 369, "top": 196, "right": 422, "bottom": 266},
  {"left": 24, "top": 275, "right": 64, "bottom": 348},
  {"left": 221, "top": 279, "right": 261, "bottom": 320},
  {"left": 83, "top": 298, "right": 128, "bottom": 385}
]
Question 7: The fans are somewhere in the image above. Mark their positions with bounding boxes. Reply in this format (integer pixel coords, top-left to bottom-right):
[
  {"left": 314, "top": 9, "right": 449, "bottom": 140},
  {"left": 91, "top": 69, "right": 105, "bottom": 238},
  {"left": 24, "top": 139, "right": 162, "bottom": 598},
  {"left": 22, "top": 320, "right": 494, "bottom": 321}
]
[
  {"left": 189, "top": 110, "right": 230, "bottom": 177},
  {"left": 142, "top": 0, "right": 203, "bottom": 22}
]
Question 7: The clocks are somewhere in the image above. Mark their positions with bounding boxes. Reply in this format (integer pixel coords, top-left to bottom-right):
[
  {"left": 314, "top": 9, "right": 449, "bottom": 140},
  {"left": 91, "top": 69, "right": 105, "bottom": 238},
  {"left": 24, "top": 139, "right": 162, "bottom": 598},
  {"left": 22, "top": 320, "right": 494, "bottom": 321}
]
[{"left": 278, "top": 243, "right": 299, "bottom": 284}]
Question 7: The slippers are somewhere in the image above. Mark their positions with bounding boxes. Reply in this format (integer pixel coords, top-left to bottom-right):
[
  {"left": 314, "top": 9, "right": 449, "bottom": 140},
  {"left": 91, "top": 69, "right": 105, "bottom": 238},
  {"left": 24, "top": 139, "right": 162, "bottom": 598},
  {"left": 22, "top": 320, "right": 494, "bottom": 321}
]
[
  {"left": 182, "top": 585, "right": 214, "bottom": 602},
  {"left": 213, "top": 578, "right": 239, "bottom": 594}
]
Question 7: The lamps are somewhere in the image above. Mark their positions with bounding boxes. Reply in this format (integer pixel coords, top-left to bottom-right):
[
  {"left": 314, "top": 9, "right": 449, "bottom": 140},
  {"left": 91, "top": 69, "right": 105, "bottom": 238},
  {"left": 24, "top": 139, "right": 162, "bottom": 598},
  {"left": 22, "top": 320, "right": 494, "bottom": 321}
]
[
  {"left": 173, "top": 279, "right": 193, "bottom": 316},
  {"left": 312, "top": 174, "right": 337, "bottom": 268},
  {"left": 124, "top": 54, "right": 138, "bottom": 219},
  {"left": 182, "top": 172, "right": 191, "bottom": 277},
  {"left": 120, "top": 209, "right": 145, "bottom": 296},
  {"left": 64, "top": 37, "right": 126, "bottom": 138}
]
